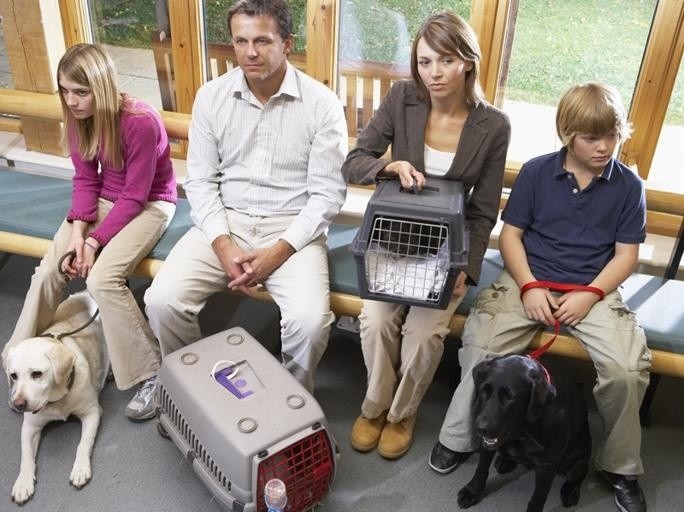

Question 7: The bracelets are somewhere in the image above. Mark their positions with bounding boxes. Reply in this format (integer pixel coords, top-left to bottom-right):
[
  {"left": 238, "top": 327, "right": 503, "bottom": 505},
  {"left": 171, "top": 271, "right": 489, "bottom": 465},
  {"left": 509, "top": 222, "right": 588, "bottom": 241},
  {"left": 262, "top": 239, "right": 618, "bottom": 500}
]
[{"left": 83, "top": 241, "right": 97, "bottom": 252}]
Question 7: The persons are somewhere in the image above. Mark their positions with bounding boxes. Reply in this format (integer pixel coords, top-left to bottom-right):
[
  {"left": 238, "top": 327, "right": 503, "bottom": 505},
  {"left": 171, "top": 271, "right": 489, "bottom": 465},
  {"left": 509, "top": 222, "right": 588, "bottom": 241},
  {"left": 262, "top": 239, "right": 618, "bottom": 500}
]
[
  {"left": 139, "top": 0, "right": 349, "bottom": 401},
  {"left": 430, "top": 80, "right": 656, "bottom": 511},
  {"left": 1, "top": 40, "right": 179, "bottom": 425},
  {"left": 337, "top": 9, "right": 512, "bottom": 462}
]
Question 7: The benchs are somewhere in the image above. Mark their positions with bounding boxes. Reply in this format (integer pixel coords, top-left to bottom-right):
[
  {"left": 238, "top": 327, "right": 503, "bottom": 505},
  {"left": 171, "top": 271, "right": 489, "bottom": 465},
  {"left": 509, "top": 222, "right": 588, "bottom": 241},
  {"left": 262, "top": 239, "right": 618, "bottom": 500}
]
[{"left": 1, "top": 169, "right": 684, "bottom": 429}]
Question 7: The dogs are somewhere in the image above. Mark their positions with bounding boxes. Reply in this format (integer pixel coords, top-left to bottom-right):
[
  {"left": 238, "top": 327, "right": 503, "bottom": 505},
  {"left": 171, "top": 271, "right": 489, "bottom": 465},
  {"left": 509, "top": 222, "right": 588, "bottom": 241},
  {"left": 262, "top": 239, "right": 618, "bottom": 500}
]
[
  {"left": 457, "top": 349, "right": 598, "bottom": 512},
  {"left": 3, "top": 287, "right": 110, "bottom": 506}
]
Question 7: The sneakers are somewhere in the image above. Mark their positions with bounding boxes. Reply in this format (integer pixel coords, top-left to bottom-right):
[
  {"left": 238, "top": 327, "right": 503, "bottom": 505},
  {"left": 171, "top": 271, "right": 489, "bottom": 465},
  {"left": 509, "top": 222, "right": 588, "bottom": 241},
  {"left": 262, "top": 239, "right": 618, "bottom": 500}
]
[
  {"left": 428, "top": 441, "right": 466, "bottom": 474},
  {"left": 125, "top": 378, "right": 156, "bottom": 420},
  {"left": 603, "top": 470, "right": 646, "bottom": 512},
  {"left": 377, "top": 412, "right": 416, "bottom": 459},
  {"left": 350, "top": 409, "right": 388, "bottom": 452}
]
